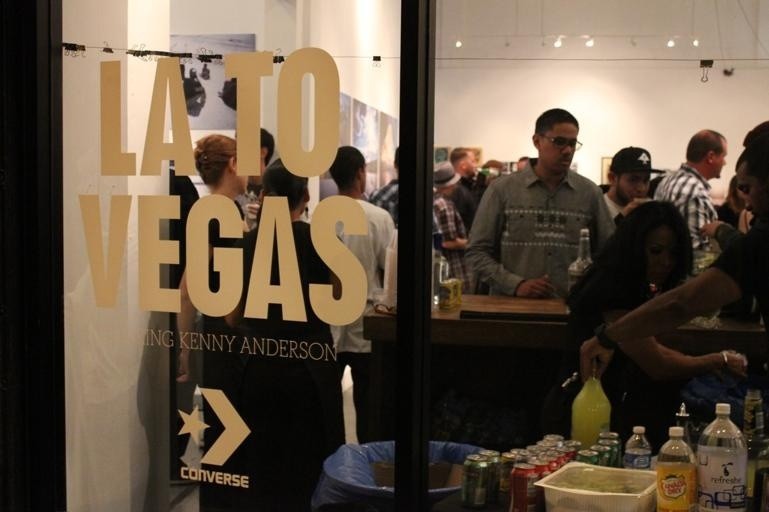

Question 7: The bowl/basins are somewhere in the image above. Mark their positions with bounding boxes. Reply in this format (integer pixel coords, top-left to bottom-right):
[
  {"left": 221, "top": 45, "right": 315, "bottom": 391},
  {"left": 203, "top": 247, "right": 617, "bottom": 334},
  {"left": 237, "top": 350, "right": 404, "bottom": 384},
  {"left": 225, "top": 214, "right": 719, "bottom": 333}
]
[{"left": 322, "top": 439, "right": 496, "bottom": 506}]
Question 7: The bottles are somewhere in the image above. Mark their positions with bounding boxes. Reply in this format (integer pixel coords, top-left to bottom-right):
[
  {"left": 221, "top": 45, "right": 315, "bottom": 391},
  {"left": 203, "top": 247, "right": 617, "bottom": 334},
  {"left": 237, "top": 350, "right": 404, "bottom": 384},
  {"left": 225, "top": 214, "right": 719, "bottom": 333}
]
[
  {"left": 655, "top": 426, "right": 699, "bottom": 512},
  {"left": 625, "top": 426, "right": 651, "bottom": 469},
  {"left": 433, "top": 249, "right": 450, "bottom": 305},
  {"left": 698, "top": 403, "right": 748, "bottom": 512},
  {"left": 476, "top": 166, "right": 502, "bottom": 178},
  {"left": 674, "top": 403, "right": 691, "bottom": 447},
  {"left": 568, "top": 229, "right": 593, "bottom": 314},
  {"left": 571, "top": 365, "right": 613, "bottom": 445},
  {"left": 743, "top": 389, "right": 764, "bottom": 459}
]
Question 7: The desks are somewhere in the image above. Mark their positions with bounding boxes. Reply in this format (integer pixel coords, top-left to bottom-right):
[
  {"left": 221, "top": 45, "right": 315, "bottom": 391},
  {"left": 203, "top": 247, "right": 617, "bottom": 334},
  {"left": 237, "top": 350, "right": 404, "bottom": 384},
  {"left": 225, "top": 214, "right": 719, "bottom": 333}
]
[{"left": 363, "top": 294, "right": 769, "bottom": 437}]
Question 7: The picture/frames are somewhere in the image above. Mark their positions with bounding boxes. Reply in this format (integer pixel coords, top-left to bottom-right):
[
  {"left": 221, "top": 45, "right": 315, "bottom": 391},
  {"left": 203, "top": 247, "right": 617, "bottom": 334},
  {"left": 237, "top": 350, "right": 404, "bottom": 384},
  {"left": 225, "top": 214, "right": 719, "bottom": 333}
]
[{"left": 600, "top": 157, "right": 614, "bottom": 185}]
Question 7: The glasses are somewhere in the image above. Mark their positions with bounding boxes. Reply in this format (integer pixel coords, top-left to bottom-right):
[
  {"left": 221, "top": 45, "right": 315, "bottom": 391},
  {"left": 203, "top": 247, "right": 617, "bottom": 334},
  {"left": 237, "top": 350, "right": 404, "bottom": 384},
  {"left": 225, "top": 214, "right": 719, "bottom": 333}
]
[{"left": 539, "top": 132, "right": 583, "bottom": 153}]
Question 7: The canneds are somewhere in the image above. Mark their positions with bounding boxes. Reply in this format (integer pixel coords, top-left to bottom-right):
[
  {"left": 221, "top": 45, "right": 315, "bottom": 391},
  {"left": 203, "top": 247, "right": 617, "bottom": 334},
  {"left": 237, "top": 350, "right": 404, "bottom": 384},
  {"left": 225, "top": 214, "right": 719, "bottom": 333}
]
[
  {"left": 449, "top": 278, "right": 462, "bottom": 305},
  {"left": 439, "top": 281, "right": 454, "bottom": 309},
  {"left": 461, "top": 432, "right": 623, "bottom": 512}
]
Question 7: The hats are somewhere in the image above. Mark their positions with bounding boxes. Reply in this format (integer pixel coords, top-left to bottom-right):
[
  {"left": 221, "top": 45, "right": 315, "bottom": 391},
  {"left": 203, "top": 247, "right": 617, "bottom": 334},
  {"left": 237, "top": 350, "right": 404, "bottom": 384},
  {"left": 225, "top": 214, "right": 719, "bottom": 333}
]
[
  {"left": 609, "top": 145, "right": 665, "bottom": 174},
  {"left": 432, "top": 160, "right": 463, "bottom": 190}
]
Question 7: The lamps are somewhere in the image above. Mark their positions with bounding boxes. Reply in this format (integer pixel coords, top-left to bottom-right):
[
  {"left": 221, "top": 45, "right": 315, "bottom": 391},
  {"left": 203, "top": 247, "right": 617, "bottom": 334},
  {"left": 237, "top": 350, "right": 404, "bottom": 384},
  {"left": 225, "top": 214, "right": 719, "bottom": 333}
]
[{"left": 454, "top": 37, "right": 702, "bottom": 50}]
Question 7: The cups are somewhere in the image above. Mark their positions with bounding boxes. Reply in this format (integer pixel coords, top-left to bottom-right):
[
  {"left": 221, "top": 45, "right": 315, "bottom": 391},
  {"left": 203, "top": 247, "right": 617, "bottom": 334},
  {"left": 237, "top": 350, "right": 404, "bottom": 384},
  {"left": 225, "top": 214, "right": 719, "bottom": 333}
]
[{"left": 382, "top": 230, "right": 398, "bottom": 305}]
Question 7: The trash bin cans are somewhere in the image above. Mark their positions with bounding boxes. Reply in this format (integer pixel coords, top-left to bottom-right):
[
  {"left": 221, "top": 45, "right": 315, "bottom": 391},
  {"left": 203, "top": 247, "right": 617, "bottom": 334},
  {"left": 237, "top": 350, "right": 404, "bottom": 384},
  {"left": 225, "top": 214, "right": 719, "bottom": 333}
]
[{"left": 323, "top": 441, "right": 487, "bottom": 512}]
